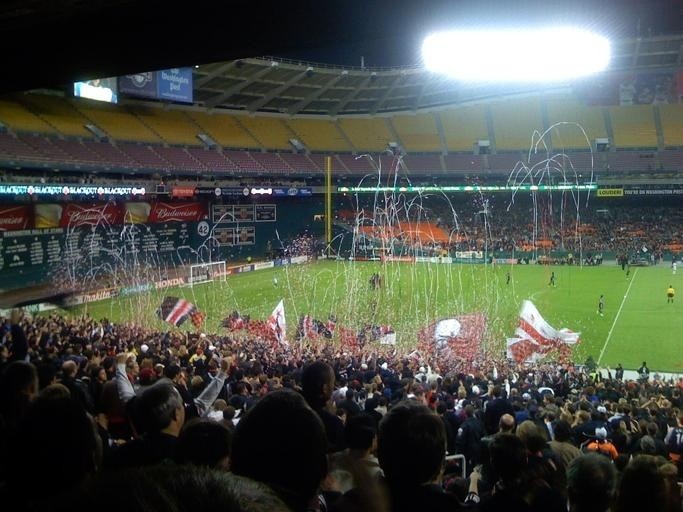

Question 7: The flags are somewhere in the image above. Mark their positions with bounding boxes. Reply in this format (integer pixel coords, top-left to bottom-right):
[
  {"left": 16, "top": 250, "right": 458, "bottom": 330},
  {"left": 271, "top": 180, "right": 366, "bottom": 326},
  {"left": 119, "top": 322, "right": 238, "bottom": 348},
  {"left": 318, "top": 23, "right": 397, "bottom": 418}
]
[
  {"left": 153, "top": 295, "right": 198, "bottom": 329},
  {"left": 505, "top": 298, "right": 581, "bottom": 364},
  {"left": 264, "top": 297, "right": 288, "bottom": 345}
]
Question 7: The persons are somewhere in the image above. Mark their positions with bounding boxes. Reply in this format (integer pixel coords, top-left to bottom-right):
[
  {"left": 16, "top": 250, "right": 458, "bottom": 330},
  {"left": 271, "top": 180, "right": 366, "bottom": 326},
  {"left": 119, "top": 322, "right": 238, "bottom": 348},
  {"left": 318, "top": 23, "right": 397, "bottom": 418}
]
[
  {"left": 596, "top": 294, "right": 606, "bottom": 316},
  {"left": 625, "top": 266, "right": 631, "bottom": 281},
  {"left": 548, "top": 272, "right": 557, "bottom": 288},
  {"left": 1, "top": 308, "right": 681, "bottom": 509},
  {"left": 368, "top": 273, "right": 384, "bottom": 289},
  {"left": 505, "top": 269, "right": 510, "bottom": 284},
  {"left": 666, "top": 285, "right": 674, "bottom": 304},
  {"left": 672, "top": 260, "right": 677, "bottom": 274},
  {"left": 284, "top": 195, "right": 682, "bottom": 269}
]
